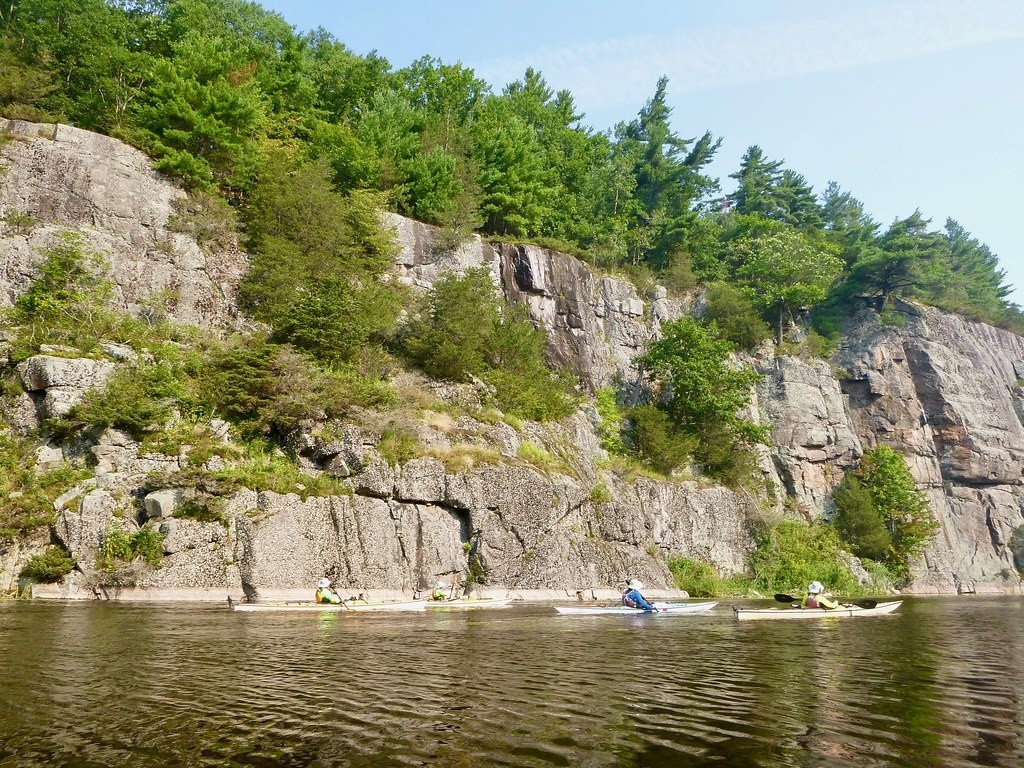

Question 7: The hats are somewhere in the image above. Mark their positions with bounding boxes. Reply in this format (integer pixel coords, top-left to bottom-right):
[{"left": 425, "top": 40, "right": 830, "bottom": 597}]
[
  {"left": 434, "top": 581, "right": 445, "bottom": 588},
  {"left": 628, "top": 579, "right": 644, "bottom": 590},
  {"left": 809, "top": 581, "right": 824, "bottom": 594},
  {"left": 319, "top": 578, "right": 331, "bottom": 587}
]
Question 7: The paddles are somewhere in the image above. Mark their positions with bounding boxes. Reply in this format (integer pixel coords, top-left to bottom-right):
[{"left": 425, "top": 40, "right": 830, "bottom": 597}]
[
  {"left": 626, "top": 580, "right": 659, "bottom": 613},
  {"left": 331, "top": 585, "right": 349, "bottom": 610},
  {"left": 449, "top": 578, "right": 458, "bottom": 597},
  {"left": 775, "top": 594, "right": 877, "bottom": 609}
]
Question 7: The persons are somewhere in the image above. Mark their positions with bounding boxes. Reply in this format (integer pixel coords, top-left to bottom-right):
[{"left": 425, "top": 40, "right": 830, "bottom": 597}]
[
  {"left": 801, "top": 581, "right": 847, "bottom": 610},
  {"left": 622, "top": 579, "right": 654, "bottom": 610},
  {"left": 315, "top": 578, "right": 364, "bottom": 604},
  {"left": 432, "top": 581, "right": 447, "bottom": 601}
]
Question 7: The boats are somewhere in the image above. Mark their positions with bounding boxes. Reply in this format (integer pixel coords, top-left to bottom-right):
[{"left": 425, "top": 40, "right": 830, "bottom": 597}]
[
  {"left": 234, "top": 595, "right": 431, "bottom": 612},
  {"left": 553, "top": 600, "right": 720, "bottom": 616},
  {"left": 737, "top": 599, "right": 904, "bottom": 620},
  {"left": 425, "top": 596, "right": 514, "bottom": 608}
]
